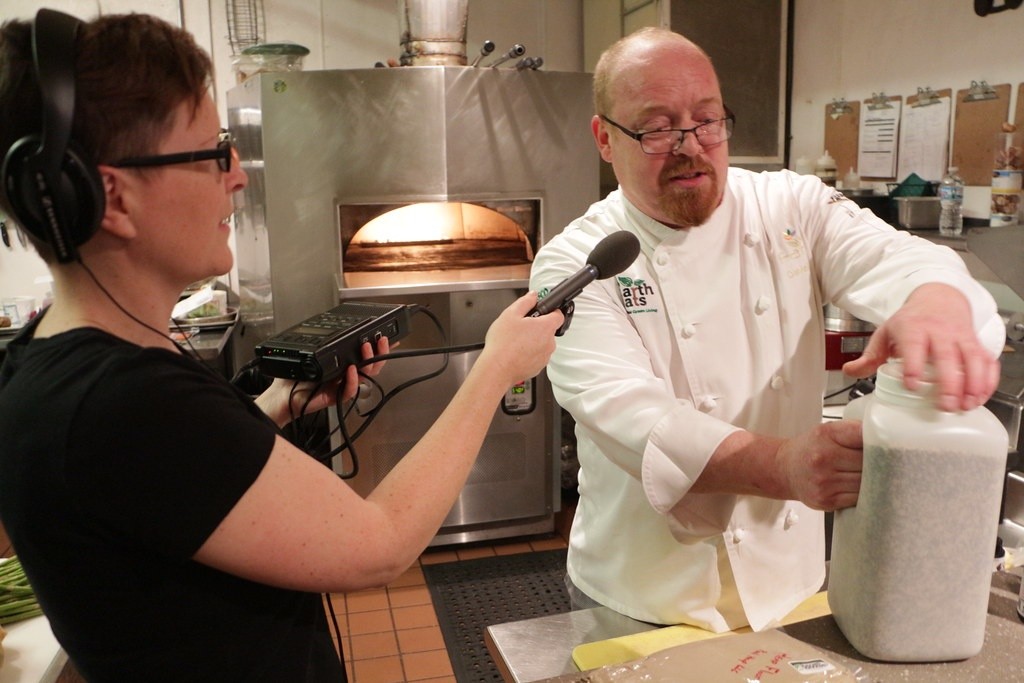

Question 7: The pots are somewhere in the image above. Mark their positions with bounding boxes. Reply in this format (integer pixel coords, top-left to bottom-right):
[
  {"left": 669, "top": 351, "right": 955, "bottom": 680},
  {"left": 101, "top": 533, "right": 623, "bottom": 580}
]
[{"left": 894, "top": 198, "right": 941, "bottom": 229}]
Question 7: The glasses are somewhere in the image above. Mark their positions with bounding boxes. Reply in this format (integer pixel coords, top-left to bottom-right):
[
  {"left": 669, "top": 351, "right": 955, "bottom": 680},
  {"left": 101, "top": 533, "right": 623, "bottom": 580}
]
[
  {"left": 109, "top": 128, "right": 236, "bottom": 173},
  {"left": 600, "top": 103, "right": 736, "bottom": 155}
]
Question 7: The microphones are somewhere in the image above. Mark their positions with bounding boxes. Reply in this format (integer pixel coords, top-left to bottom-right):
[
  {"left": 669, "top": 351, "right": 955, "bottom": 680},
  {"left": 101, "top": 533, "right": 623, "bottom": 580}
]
[{"left": 528, "top": 230, "right": 640, "bottom": 317}]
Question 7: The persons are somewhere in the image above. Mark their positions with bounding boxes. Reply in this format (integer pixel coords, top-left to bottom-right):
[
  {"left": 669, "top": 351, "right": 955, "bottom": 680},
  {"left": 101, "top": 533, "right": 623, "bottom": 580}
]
[
  {"left": 525, "top": 25, "right": 1006, "bottom": 635},
  {"left": 0, "top": 11, "right": 573, "bottom": 683}
]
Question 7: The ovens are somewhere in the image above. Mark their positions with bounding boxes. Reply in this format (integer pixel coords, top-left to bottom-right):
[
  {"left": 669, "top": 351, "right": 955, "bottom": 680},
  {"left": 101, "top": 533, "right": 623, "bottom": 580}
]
[{"left": 225, "top": 65, "right": 601, "bottom": 548}]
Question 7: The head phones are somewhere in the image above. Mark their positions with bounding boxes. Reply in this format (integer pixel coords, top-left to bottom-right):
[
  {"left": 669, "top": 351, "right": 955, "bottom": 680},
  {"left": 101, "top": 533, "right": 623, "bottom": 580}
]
[{"left": 0, "top": 7, "right": 108, "bottom": 264}]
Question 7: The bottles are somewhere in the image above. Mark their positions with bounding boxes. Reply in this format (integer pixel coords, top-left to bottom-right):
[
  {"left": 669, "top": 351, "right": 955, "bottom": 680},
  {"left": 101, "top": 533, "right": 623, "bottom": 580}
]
[
  {"left": 815, "top": 150, "right": 836, "bottom": 189},
  {"left": 938, "top": 168, "right": 964, "bottom": 238},
  {"left": 826, "top": 357, "right": 1009, "bottom": 662},
  {"left": 844, "top": 166, "right": 860, "bottom": 190}
]
